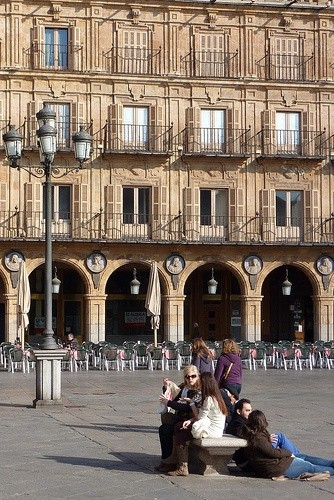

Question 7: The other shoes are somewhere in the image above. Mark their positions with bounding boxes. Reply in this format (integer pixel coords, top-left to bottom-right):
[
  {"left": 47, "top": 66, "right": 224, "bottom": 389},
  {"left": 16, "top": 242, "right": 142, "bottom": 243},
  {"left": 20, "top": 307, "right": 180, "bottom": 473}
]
[{"left": 155, "top": 458, "right": 177, "bottom": 472}]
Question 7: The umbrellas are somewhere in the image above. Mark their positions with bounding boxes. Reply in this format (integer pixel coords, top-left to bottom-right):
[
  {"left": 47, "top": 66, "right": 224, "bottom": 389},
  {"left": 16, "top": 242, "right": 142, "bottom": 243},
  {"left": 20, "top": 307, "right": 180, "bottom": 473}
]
[
  {"left": 16, "top": 260, "right": 30, "bottom": 349},
  {"left": 145, "top": 261, "right": 161, "bottom": 348}
]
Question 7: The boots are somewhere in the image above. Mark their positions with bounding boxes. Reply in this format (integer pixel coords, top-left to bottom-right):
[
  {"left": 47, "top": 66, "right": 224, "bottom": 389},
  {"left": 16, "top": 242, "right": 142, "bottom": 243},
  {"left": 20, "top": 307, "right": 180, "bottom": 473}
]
[
  {"left": 163, "top": 435, "right": 185, "bottom": 466},
  {"left": 168, "top": 446, "right": 189, "bottom": 476}
]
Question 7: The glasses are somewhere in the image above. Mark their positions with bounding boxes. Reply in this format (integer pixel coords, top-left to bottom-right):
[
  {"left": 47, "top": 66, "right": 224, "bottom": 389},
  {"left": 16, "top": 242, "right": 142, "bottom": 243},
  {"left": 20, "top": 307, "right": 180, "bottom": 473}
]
[{"left": 185, "top": 374, "right": 197, "bottom": 379}]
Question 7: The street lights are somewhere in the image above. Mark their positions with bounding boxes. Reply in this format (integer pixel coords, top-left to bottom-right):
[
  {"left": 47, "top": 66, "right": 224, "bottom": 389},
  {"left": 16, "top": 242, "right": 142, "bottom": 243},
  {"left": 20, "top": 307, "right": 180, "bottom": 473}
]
[{"left": 2, "top": 102, "right": 95, "bottom": 410}]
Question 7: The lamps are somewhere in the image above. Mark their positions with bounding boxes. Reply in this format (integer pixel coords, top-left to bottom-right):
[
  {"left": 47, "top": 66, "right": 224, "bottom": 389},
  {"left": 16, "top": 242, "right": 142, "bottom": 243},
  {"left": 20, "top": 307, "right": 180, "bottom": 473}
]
[
  {"left": 207, "top": 266, "right": 218, "bottom": 294},
  {"left": 52, "top": 264, "right": 61, "bottom": 294},
  {"left": 130, "top": 266, "right": 141, "bottom": 294},
  {"left": 280, "top": 267, "right": 292, "bottom": 295}
]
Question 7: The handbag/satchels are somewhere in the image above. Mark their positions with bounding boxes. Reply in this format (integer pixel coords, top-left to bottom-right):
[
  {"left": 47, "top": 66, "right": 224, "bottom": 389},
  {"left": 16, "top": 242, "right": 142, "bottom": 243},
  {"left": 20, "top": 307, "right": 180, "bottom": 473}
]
[{"left": 272, "top": 471, "right": 329, "bottom": 482}]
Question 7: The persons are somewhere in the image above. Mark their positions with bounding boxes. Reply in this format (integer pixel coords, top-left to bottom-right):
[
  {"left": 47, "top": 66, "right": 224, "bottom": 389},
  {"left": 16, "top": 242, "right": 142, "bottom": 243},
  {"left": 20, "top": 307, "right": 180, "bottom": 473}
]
[
  {"left": 8, "top": 255, "right": 22, "bottom": 269},
  {"left": 63, "top": 333, "right": 83, "bottom": 371},
  {"left": 90, "top": 255, "right": 104, "bottom": 271},
  {"left": 169, "top": 258, "right": 182, "bottom": 272},
  {"left": 219, "top": 388, "right": 334, "bottom": 478},
  {"left": 192, "top": 323, "right": 202, "bottom": 340},
  {"left": 213, "top": 339, "right": 242, "bottom": 399},
  {"left": 161, "top": 371, "right": 228, "bottom": 476},
  {"left": 319, "top": 258, "right": 332, "bottom": 273},
  {"left": 155, "top": 365, "right": 203, "bottom": 471},
  {"left": 246, "top": 257, "right": 261, "bottom": 274},
  {"left": 191, "top": 338, "right": 213, "bottom": 373}
]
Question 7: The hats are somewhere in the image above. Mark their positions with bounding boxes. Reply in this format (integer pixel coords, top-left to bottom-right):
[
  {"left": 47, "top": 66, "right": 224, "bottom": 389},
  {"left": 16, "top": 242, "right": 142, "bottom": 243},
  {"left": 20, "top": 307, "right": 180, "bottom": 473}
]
[{"left": 226, "top": 387, "right": 239, "bottom": 400}]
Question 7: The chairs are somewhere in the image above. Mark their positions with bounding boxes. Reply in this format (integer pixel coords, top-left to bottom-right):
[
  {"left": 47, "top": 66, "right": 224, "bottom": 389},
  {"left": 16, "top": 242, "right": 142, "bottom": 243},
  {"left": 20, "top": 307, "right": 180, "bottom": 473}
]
[
  {"left": 62, "top": 340, "right": 334, "bottom": 373},
  {"left": 0, "top": 342, "right": 36, "bottom": 374}
]
